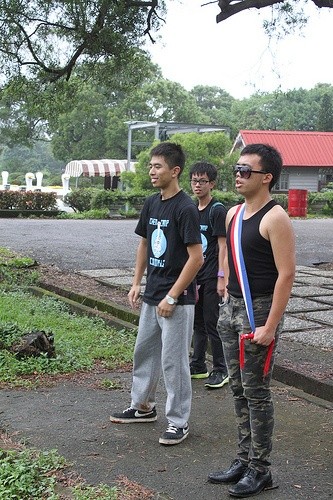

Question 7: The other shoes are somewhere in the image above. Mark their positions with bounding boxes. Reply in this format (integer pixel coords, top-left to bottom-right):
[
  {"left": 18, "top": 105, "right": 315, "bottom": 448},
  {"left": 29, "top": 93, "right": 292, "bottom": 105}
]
[
  {"left": 110, "top": 406, "right": 158, "bottom": 424},
  {"left": 159, "top": 423, "right": 190, "bottom": 445}
]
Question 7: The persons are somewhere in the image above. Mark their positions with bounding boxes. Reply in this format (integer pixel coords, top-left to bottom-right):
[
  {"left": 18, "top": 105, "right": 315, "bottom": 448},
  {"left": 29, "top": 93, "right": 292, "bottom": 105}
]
[
  {"left": 190, "top": 162, "right": 229, "bottom": 388},
  {"left": 109, "top": 143, "right": 204, "bottom": 444},
  {"left": 208, "top": 144, "right": 296, "bottom": 498}
]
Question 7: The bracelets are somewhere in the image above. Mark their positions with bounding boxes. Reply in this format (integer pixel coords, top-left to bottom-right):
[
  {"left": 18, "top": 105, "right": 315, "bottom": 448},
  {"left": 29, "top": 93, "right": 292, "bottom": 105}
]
[{"left": 217, "top": 271, "right": 225, "bottom": 277}]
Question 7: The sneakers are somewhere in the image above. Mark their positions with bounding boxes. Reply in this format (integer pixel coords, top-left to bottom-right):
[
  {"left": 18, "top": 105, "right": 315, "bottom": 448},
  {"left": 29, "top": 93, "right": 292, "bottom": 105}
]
[
  {"left": 190, "top": 368, "right": 209, "bottom": 378},
  {"left": 204, "top": 369, "right": 229, "bottom": 388}
]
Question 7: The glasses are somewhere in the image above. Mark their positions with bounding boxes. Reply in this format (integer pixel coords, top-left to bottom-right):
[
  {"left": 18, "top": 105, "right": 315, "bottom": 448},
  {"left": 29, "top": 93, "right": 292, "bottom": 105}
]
[
  {"left": 233, "top": 164, "right": 267, "bottom": 179},
  {"left": 189, "top": 179, "right": 210, "bottom": 185}
]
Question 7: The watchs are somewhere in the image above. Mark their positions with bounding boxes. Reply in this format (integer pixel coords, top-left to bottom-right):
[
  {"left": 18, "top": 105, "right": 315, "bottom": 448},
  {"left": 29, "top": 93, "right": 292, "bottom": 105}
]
[{"left": 165, "top": 295, "right": 178, "bottom": 305}]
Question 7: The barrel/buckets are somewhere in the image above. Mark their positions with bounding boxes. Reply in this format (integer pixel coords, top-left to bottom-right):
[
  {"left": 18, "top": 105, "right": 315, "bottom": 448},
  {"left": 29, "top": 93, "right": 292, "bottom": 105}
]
[{"left": 288, "top": 189, "right": 307, "bottom": 217}]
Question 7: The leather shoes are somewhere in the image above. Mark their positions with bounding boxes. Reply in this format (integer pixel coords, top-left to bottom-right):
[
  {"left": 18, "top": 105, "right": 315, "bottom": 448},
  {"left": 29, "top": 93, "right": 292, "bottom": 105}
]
[{"left": 208, "top": 459, "right": 272, "bottom": 498}]
